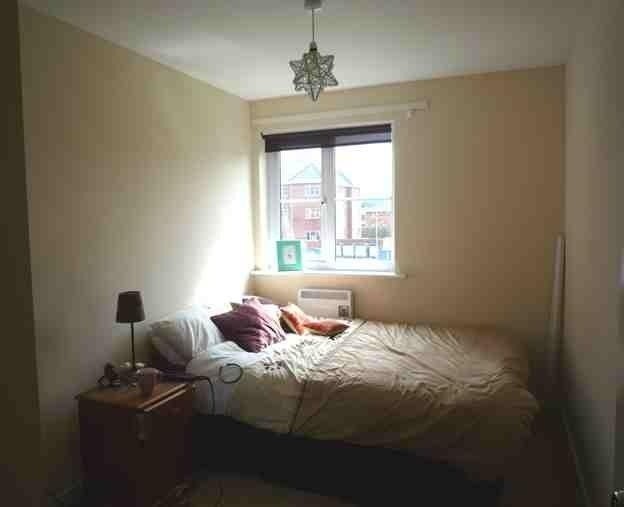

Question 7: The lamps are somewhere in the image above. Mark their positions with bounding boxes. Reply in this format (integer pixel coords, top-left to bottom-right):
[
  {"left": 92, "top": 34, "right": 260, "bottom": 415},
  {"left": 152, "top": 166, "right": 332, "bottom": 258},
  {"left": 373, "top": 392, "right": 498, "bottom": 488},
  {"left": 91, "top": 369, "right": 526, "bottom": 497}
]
[
  {"left": 113, "top": 291, "right": 148, "bottom": 380},
  {"left": 288, "top": 0, "right": 338, "bottom": 102}
]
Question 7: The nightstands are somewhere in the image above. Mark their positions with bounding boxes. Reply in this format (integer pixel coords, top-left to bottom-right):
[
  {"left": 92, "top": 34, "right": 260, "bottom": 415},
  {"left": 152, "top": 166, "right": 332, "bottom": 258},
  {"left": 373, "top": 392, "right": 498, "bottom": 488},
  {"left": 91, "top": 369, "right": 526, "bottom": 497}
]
[{"left": 75, "top": 380, "right": 194, "bottom": 507}]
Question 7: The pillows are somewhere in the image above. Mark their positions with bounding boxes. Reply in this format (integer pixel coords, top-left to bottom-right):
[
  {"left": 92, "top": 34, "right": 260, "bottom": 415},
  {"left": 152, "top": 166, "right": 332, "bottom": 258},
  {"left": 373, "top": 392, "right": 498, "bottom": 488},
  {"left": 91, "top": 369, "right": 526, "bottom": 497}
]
[
  {"left": 143, "top": 310, "right": 186, "bottom": 364},
  {"left": 153, "top": 301, "right": 237, "bottom": 356},
  {"left": 302, "top": 320, "right": 348, "bottom": 338},
  {"left": 210, "top": 297, "right": 287, "bottom": 353},
  {"left": 278, "top": 305, "right": 305, "bottom": 338}
]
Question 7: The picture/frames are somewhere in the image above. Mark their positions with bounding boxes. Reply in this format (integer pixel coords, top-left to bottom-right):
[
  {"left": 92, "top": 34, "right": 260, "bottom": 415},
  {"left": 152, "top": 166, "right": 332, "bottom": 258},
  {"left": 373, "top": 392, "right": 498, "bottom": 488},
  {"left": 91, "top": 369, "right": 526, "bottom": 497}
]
[{"left": 277, "top": 240, "right": 302, "bottom": 270}]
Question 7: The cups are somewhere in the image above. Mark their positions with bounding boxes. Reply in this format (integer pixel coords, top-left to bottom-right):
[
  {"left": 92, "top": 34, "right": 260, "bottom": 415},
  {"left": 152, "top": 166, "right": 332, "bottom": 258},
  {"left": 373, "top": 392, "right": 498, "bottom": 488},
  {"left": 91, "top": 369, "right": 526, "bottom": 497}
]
[{"left": 136, "top": 367, "right": 159, "bottom": 395}]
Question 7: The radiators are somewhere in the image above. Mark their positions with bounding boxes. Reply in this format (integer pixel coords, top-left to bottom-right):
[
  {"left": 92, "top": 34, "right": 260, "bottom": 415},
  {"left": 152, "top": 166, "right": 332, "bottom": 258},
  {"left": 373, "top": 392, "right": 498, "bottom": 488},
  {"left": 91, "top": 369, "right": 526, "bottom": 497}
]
[{"left": 297, "top": 290, "right": 355, "bottom": 319}]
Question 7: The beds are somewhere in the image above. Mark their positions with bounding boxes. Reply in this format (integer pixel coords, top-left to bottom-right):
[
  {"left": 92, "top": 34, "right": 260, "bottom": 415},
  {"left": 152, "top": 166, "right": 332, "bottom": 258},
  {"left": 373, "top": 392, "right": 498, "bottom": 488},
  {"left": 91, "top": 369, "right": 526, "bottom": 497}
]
[{"left": 151, "top": 292, "right": 542, "bottom": 506}]
